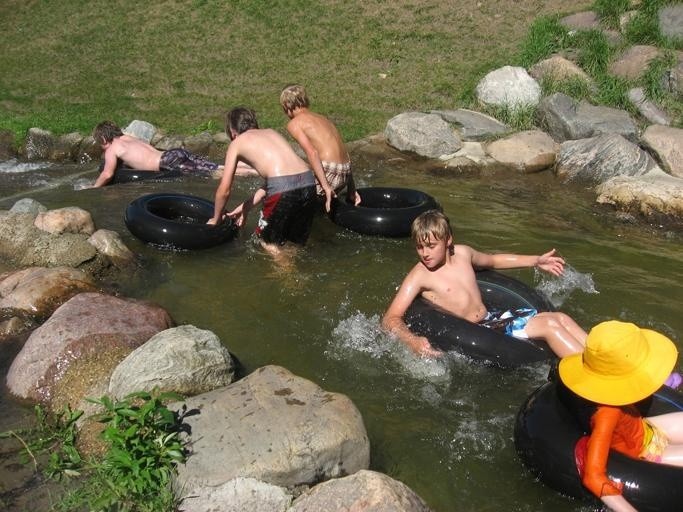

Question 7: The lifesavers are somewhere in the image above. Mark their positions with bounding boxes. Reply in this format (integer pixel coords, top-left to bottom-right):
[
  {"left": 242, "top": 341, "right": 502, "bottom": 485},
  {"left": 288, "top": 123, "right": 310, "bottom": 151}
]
[
  {"left": 328, "top": 187, "right": 443, "bottom": 238},
  {"left": 404, "top": 270, "right": 557, "bottom": 366},
  {"left": 98, "top": 148, "right": 183, "bottom": 183},
  {"left": 514, "top": 377, "right": 683, "bottom": 512},
  {"left": 124, "top": 192, "right": 236, "bottom": 250}
]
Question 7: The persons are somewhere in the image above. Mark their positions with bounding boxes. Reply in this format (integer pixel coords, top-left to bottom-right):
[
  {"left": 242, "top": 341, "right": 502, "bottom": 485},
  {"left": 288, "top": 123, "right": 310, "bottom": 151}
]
[
  {"left": 558, "top": 320, "right": 683, "bottom": 512},
  {"left": 380, "top": 208, "right": 588, "bottom": 360},
  {"left": 279, "top": 85, "right": 362, "bottom": 214},
  {"left": 80, "top": 121, "right": 258, "bottom": 190},
  {"left": 206, "top": 107, "right": 317, "bottom": 278}
]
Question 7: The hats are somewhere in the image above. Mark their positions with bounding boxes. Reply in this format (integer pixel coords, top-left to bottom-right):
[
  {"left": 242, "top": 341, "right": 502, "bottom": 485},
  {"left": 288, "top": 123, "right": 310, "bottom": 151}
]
[{"left": 555, "top": 316, "right": 680, "bottom": 410}]
